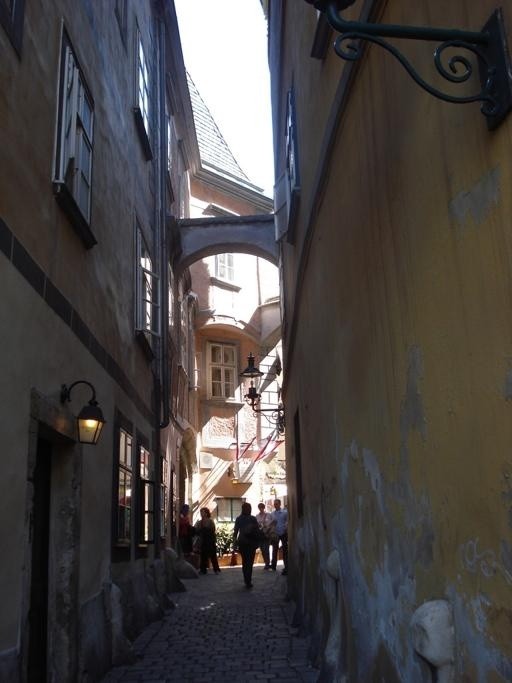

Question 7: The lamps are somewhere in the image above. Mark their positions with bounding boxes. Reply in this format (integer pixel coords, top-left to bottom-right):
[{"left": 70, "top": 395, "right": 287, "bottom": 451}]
[
  {"left": 239, "top": 350, "right": 284, "bottom": 411},
  {"left": 60, "top": 379, "right": 105, "bottom": 444}
]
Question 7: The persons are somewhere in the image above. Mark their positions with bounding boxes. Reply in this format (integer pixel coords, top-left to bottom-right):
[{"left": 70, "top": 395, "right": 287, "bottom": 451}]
[
  {"left": 255, "top": 502, "right": 272, "bottom": 569},
  {"left": 178, "top": 503, "right": 196, "bottom": 558},
  {"left": 232, "top": 502, "right": 260, "bottom": 588},
  {"left": 193, "top": 507, "right": 221, "bottom": 575},
  {"left": 267, "top": 498, "right": 289, "bottom": 574}
]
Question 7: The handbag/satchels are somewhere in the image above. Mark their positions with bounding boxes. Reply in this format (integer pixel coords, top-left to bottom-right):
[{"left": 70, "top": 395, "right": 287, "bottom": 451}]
[{"left": 245, "top": 522, "right": 257, "bottom": 535}]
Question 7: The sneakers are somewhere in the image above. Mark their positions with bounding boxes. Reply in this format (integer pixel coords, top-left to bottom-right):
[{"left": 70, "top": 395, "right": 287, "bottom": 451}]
[
  {"left": 198, "top": 566, "right": 221, "bottom": 574},
  {"left": 264, "top": 561, "right": 277, "bottom": 570}
]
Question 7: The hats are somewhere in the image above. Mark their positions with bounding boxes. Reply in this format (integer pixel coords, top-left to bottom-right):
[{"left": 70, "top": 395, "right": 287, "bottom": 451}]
[{"left": 182, "top": 505, "right": 189, "bottom": 512}]
